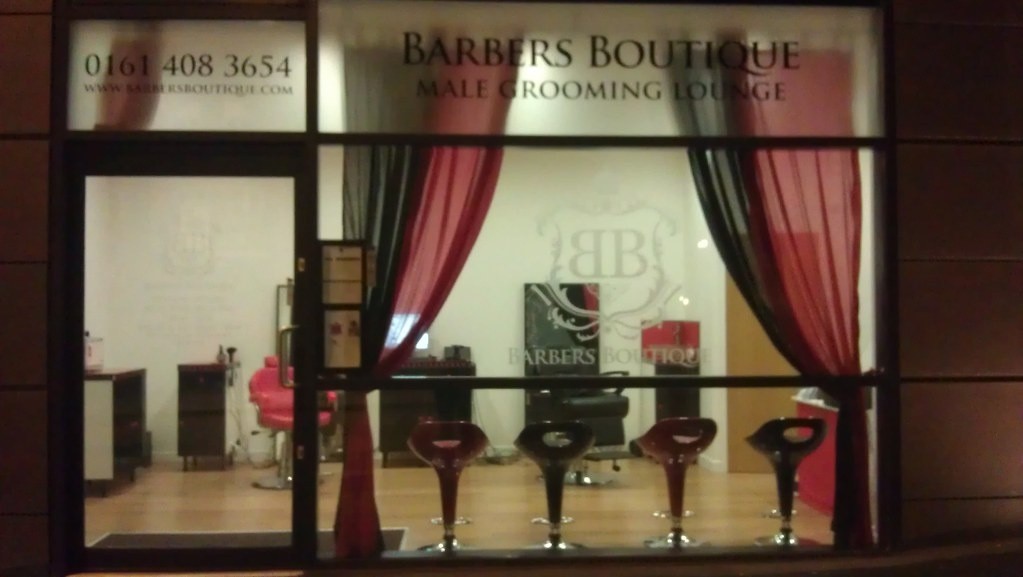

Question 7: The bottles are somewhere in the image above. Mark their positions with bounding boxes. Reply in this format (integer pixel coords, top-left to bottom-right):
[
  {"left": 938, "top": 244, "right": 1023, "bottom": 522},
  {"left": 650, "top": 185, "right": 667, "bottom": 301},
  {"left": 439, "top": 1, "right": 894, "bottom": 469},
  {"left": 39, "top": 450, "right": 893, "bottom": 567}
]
[{"left": 217, "top": 346, "right": 226, "bottom": 363}]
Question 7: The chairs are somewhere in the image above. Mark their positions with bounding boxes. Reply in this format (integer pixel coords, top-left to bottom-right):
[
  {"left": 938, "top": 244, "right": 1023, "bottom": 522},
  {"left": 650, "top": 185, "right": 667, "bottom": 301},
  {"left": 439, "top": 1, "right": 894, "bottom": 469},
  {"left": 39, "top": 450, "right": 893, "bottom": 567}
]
[
  {"left": 538, "top": 371, "right": 629, "bottom": 485},
  {"left": 248, "top": 355, "right": 337, "bottom": 490}
]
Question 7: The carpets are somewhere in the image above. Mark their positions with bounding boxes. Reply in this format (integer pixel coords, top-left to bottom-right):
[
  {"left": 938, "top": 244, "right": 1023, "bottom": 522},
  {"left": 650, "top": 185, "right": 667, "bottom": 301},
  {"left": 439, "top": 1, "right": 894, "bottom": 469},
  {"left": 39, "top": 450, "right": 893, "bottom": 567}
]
[{"left": 90, "top": 527, "right": 409, "bottom": 551}]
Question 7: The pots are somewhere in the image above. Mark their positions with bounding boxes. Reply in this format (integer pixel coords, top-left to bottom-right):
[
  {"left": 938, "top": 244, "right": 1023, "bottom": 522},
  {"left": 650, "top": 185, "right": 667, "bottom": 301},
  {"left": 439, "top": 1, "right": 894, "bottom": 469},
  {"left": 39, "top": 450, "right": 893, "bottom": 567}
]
[{"left": 84, "top": 332, "right": 105, "bottom": 372}]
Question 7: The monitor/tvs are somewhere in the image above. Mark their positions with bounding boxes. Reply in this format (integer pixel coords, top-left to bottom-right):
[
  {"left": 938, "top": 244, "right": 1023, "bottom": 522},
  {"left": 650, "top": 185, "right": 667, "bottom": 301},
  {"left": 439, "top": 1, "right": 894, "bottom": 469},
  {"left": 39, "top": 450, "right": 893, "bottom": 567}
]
[{"left": 384, "top": 314, "right": 430, "bottom": 360}]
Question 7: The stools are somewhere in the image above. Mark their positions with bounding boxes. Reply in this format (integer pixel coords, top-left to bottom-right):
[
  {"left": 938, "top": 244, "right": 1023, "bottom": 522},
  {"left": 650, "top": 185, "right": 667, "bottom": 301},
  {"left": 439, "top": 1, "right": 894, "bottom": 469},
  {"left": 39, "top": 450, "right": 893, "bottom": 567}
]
[
  {"left": 634, "top": 418, "right": 718, "bottom": 551},
  {"left": 406, "top": 420, "right": 489, "bottom": 551},
  {"left": 745, "top": 417, "right": 828, "bottom": 547},
  {"left": 514, "top": 421, "right": 595, "bottom": 548}
]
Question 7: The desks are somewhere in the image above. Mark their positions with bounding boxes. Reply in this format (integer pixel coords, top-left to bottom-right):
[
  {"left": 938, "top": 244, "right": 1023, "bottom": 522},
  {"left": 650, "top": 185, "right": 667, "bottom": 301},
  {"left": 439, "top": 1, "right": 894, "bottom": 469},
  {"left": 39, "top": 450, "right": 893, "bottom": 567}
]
[{"left": 83, "top": 368, "right": 147, "bottom": 498}]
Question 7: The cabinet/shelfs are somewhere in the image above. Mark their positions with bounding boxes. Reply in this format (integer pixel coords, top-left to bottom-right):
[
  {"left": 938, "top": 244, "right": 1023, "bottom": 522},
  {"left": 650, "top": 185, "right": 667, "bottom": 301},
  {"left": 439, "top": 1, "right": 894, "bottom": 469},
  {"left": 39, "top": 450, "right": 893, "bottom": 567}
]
[
  {"left": 378, "top": 362, "right": 477, "bottom": 468},
  {"left": 176, "top": 361, "right": 242, "bottom": 471}
]
